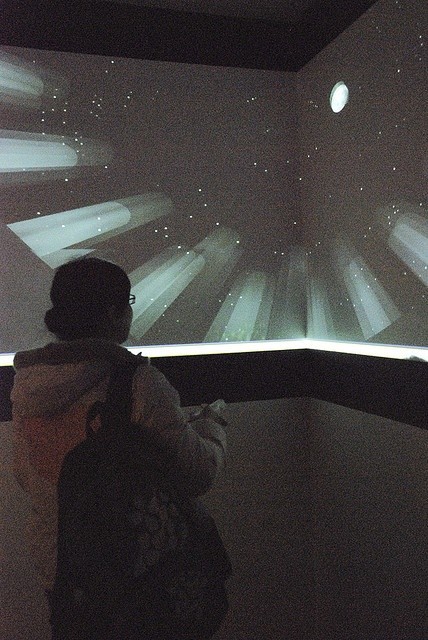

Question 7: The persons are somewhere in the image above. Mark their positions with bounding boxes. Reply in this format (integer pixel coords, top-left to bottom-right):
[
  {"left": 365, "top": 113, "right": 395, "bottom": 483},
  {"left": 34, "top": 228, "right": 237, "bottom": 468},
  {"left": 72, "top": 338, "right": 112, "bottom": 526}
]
[{"left": 9, "top": 257, "right": 227, "bottom": 640}]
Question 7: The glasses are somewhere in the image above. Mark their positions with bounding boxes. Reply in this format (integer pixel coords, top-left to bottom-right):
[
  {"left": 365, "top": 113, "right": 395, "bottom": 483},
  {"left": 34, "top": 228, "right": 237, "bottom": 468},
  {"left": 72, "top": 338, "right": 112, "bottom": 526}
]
[{"left": 125, "top": 294, "right": 134, "bottom": 304}]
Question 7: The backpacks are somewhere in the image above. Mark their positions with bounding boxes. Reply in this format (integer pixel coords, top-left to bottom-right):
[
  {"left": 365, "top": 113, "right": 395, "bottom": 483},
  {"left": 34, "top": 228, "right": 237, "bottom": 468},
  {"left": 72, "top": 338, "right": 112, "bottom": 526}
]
[{"left": 44, "top": 357, "right": 231, "bottom": 640}]
